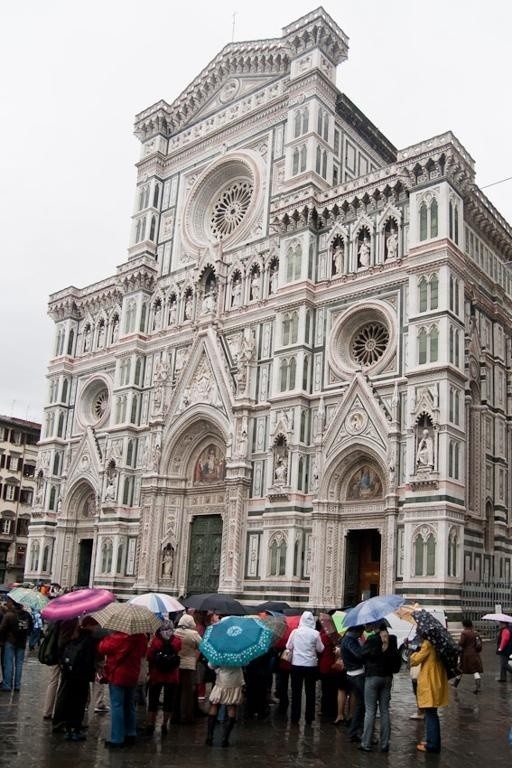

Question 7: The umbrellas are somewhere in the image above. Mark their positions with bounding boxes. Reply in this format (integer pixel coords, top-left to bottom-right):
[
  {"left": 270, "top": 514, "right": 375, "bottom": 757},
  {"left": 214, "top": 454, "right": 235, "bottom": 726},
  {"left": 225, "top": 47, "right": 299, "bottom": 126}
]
[{"left": 481, "top": 613, "right": 512, "bottom": 624}]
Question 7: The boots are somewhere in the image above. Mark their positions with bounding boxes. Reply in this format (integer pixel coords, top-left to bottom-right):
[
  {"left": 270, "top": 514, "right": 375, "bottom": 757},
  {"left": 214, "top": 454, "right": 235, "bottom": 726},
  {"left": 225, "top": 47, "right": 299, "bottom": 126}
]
[
  {"left": 221, "top": 716, "right": 235, "bottom": 748},
  {"left": 205, "top": 713, "right": 218, "bottom": 746},
  {"left": 451, "top": 678, "right": 460, "bottom": 688},
  {"left": 473, "top": 678, "right": 480, "bottom": 695}
]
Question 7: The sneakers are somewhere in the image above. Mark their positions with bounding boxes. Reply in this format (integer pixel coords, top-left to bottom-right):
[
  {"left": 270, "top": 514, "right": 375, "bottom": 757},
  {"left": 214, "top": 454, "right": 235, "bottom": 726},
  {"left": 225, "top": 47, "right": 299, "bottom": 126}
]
[{"left": 95, "top": 706, "right": 111, "bottom": 712}]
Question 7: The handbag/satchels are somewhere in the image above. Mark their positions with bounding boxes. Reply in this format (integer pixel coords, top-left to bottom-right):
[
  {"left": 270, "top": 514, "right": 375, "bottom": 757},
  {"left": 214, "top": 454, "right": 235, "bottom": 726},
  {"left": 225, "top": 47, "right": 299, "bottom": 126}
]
[
  {"left": 280, "top": 649, "right": 292, "bottom": 671},
  {"left": 138, "top": 658, "right": 151, "bottom": 686},
  {"left": 332, "top": 657, "right": 344, "bottom": 672},
  {"left": 96, "top": 671, "right": 107, "bottom": 685},
  {"left": 475, "top": 633, "right": 482, "bottom": 651}
]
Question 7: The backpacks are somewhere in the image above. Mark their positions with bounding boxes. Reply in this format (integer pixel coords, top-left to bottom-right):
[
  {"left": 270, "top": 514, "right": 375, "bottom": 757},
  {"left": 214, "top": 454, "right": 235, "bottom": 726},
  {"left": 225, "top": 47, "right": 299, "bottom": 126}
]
[
  {"left": 38, "top": 621, "right": 68, "bottom": 667},
  {"left": 57, "top": 636, "right": 90, "bottom": 680},
  {"left": 150, "top": 634, "right": 180, "bottom": 675}
]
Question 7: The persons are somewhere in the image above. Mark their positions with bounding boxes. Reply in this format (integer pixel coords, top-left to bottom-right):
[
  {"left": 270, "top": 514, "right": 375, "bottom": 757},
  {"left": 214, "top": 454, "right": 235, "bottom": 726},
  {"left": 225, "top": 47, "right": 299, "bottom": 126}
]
[
  {"left": 208, "top": 450, "right": 216, "bottom": 471},
  {"left": 152, "top": 444, "right": 161, "bottom": 469},
  {"left": 204, "top": 662, "right": 244, "bottom": 747},
  {"left": 239, "top": 429, "right": 248, "bottom": 459},
  {"left": 269, "top": 266, "right": 278, "bottom": 294},
  {"left": 98, "top": 631, "right": 147, "bottom": 748},
  {"left": 232, "top": 279, "right": 242, "bottom": 307},
  {"left": 274, "top": 450, "right": 289, "bottom": 482},
  {"left": 87, "top": 496, "right": 97, "bottom": 517},
  {"left": 409, "top": 635, "right": 450, "bottom": 754},
  {"left": 154, "top": 305, "right": 162, "bottom": 329},
  {"left": 495, "top": 619, "right": 512, "bottom": 682},
  {"left": 201, "top": 286, "right": 217, "bottom": 313},
  {"left": 112, "top": 320, "right": 119, "bottom": 343},
  {"left": 360, "top": 622, "right": 397, "bottom": 753},
  {"left": 168, "top": 300, "right": 178, "bottom": 324},
  {"left": 224, "top": 432, "right": 233, "bottom": 461},
  {"left": 99, "top": 325, "right": 104, "bottom": 348},
  {"left": 385, "top": 228, "right": 398, "bottom": 258},
  {"left": 251, "top": 273, "right": 261, "bottom": 299},
  {"left": 1, "top": 580, "right": 365, "bottom": 735},
  {"left": 332, "top": 246, "right": 342, "bottom": 273},
  {"left": 85, "top": 329, "right": 92, "bottom": 352},
  {"left": 358, "top": 236, "right": 371, "bottom": 266},
  {"left": 162, "top": 548, "right": 174, "bottom": 577},
  {"left": 184, "top": 295, "right": 193, "bottom": 320},
  {"left": 450, "top": 618, "right": 484, "bottom": 693},
  {"left": 107, "top": 473, "right": 115, "bottom": 500},
  {"left": 62, "top": 617, "right": 100, "bottom": 741},
  {"left": 415, "top": 428, "right": 434, "bottom": 469}
]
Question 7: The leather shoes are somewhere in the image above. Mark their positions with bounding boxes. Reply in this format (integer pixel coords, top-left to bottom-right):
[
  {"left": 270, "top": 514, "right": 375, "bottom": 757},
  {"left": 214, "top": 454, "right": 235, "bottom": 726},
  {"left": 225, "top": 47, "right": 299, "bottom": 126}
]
[
  {"left": 417, "top": 744, "right": 427, "bottom": 751},
  {"left": 410, "top": 713, "right": 424, "bottom": 719}
]
